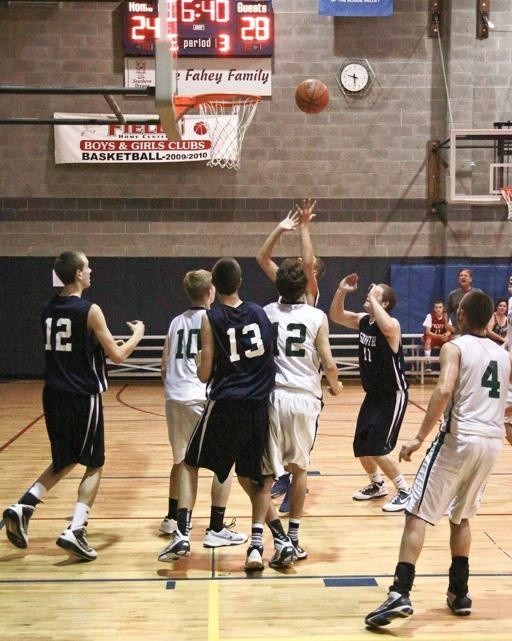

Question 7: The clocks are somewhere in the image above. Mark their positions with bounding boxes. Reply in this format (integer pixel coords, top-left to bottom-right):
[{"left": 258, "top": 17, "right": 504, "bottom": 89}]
[{"left": 335, "top": 56, "right": 377, "bottom": 97}]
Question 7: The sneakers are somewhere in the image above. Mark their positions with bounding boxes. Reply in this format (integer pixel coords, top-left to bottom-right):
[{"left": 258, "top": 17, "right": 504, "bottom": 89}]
[
  {"left": 2, "top": 500, "right": 36, "bottom": 550},
  {"left": 381, "top": 488, "right": 412, "bottom": 513},
  {"left": 279, "top": 487, "right": 308, "bottom": 513},
  {"left": 157, "top": 533, "right": 192, "bottom": 564},
  {"left": 158, "top": 515, "right": 193, "bottom": 535},
  {"left": 244, "top": 544, "right": 264, "bottom": 571},
  {"left": 351, "top": 480, "right": 389, "bottom": 502},
  {"left": 55, "top": 523, "right": 98, "bottom": 562},
  {"left": 268, "top": 536, "right": 298, "bottom": 569},
  {"left": 424, "top": 362, "right": 432, "bottom": 372},
  {"left": 364, "top": 586, "right": 414, "bottom": 628},
  {"left": 290, "top": 537, "right": 308, "bottom": 559},
  {"left": 202, "top": 522, "right": 249, "bottom": 548},
  {"left": 270, "top": 475, "right": 290, "bottom": 500},
  {"left": 446, "top": 587, "right": 474, "bottom": 616}
]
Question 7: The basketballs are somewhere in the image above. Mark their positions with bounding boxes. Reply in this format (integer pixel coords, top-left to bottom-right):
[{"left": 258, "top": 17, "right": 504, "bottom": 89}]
[
  {"left": 296, "top": 80, "right": 329, "bottom": 113},
  {"left": 194, "top": 121, "right": 210, "bottom": 134}
]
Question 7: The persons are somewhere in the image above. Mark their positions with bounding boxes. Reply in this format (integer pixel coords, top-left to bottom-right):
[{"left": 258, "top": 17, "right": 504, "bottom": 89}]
[
  {"left": 256, "top": 199, "right": 326, "bottom": 514},
  {"left": 328, "top": 273, "right": 413, "bottom": 512},
  {"left": 365, "top": 290, "right": 512, "bottom": 628},
  {"left": 244, "top": 257, "right": 344, "bottom": 570},
  {"left": 421, "top": 267, "right": 512, "bottom": 446},
  {"left": 158, "top": 268, "right": 249, "bottom": 548},
  {"left": 157, "top": 258, "right": 298, "bottom": 567},
  {"left": 2, "top": 250, "right": 146, "bottom": 561}
]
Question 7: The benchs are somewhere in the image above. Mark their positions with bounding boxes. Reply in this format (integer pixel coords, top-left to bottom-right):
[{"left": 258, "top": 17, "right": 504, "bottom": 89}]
[{"left": 92, "top": 333, "right": 442, "bottom": 379}]
